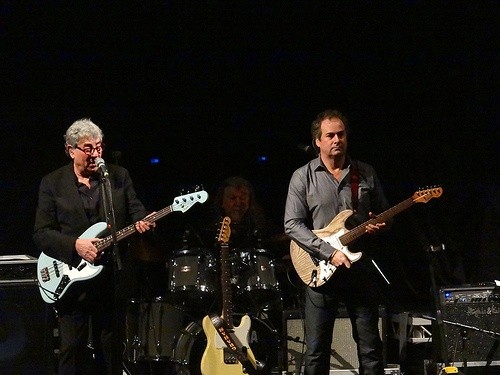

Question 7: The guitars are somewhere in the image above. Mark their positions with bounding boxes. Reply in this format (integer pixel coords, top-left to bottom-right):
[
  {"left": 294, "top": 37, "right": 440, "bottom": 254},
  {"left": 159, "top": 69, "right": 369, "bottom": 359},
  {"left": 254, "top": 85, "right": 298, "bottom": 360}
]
[
  {"left": 36, "top": 182, "right": 210, "bottom": 304},
  {"left": 200, "top": 216, "right": 256, "bottom": 375},
  {"left": 289, "top": 184, "right": 445, "bottom": 288}
]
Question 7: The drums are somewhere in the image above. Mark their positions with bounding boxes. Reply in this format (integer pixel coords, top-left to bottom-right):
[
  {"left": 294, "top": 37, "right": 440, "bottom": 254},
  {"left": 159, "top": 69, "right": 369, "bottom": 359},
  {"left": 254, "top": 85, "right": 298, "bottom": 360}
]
[
  {"left": 168, "top": 248, "right": 217, "bottom": 308},
  {"left": 130, "top": 296, "right": 191, "bottom": 367},
  {"left": 176, "top": 314, "right": 281, "bottom": 375},
  {"left": 229, "top": 249, "right": 283, "bottom": 309}
]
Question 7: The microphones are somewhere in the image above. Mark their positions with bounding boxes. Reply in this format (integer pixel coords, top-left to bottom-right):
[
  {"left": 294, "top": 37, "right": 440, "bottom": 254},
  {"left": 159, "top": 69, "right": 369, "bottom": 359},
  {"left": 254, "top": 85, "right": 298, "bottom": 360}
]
[{"left": 95, "top": 158, "right": 109, "bottom": 177}]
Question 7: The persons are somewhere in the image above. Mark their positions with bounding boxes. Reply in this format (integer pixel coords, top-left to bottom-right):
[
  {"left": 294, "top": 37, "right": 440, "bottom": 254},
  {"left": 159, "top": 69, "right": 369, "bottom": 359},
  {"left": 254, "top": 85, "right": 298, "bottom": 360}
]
[
  {"left": 283, "top": 109, "right": 392, "bottom": 375},
  {"left": 213, "top": 177, "right": 267, "bottom": 251},
  {"left": 33, "top": 119, "right": 156, "bottom": 375}
]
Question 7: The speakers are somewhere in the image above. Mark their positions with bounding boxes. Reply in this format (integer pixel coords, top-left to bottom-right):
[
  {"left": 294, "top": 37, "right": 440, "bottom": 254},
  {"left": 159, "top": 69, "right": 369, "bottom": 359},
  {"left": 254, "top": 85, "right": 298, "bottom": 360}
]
[
  {"left": 282, "top": 308, "right": 385, "bottom": 375},
  {"left": 436, "top": 286, "right": 500, "bottom": 364},
  {"left": 0, "top": 283, "right": 56, "bottom": 375}
]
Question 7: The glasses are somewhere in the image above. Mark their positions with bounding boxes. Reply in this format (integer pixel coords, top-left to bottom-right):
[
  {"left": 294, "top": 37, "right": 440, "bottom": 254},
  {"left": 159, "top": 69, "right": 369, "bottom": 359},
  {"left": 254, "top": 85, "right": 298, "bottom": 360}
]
[{"left": 76, "top": 143, "right": 105, "bottom": 155}]
